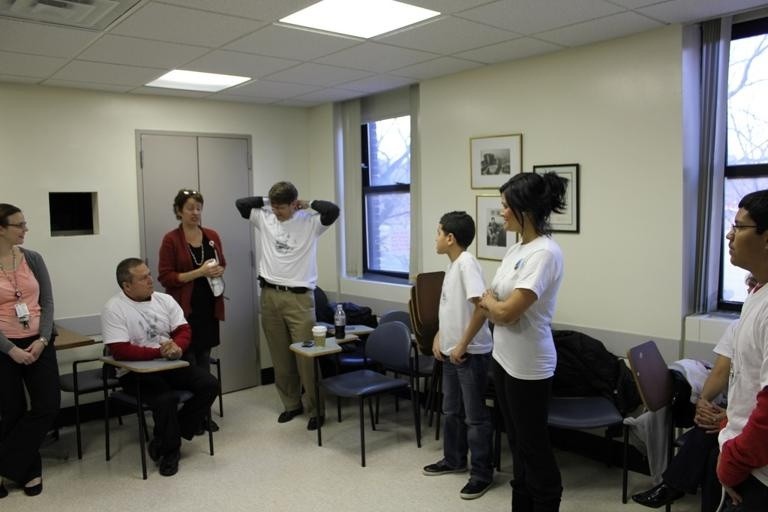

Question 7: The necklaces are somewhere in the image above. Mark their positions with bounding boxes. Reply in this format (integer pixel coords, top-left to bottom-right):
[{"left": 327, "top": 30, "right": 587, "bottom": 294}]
[
  {"left": 0, "top": 249, "right": 22, "bottom": 300},
  {"left": 187, "top": 230, "right": 205, "bottom": 266}
]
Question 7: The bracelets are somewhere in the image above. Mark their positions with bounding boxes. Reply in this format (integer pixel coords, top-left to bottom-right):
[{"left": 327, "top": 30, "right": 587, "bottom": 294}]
[{"left": 38, "top": 336, "right": 49, "bottom": 347}]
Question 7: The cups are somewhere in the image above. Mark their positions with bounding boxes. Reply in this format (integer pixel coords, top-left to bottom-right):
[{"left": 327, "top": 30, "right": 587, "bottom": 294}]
[{"left": 312, "top": 326, "right": 328, "bottom": 350}]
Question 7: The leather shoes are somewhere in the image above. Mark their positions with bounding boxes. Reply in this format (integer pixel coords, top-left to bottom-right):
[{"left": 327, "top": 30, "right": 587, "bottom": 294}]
[
  {"left": 198, "top": 418, "right": 218, "bottom": 432},
  {"left": 308, "top": 415, "right": 324, "bottom": 429},
  {"left": 149, "top": 437, "right": 180, "bottom": 476},
  {"left": 633, "top": 481, "right": 685, "bottom": 508},
  {"left": 278, "top": 406, "right": 303, "bottom": 422},
  {"left": 24, "top": 477, "right": 42, "bottom": 496}
]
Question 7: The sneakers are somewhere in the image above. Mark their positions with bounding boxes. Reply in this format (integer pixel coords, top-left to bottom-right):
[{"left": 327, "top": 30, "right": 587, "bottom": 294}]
[
  {"left": 460, "top": 478, "right": 492, "bottom": 498},
  {"left": 424, "top": 459, "right": 468, "bottom": 475}
]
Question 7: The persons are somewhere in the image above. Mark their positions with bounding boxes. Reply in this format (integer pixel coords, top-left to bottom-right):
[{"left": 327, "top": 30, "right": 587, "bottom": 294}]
[
  {"left": 421, "top": 211, "right": 494, "bottom": 500},
  {"left": 99, "top": 258, "right": 220, "bottom": 477},
  {"left": 0, "top": 352, "right": 43, "bottom": 499},
  {"left": 631, "top": 276, "right": 758, "bottom": 508},
  {"left": 475, "top": 171, "right": 569, "bottom": 512},
  {"left": 157, "top": 188, "right": 227, "bottom": 431},
  {"left": 701, "top": 189, "right": 768, "bottom": 512},
  {"left": 234, "top": 181, "right": 341, "bottom": 431},
  {"left": 1, "top": 203, "right": 61, "bottom": 444}
]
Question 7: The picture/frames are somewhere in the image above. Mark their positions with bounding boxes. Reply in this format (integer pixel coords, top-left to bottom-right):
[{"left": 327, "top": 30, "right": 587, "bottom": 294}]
[
  {"left": 470, "top": 133, "right": 523, "bottom": 190},
  {"left": 475, "top": 195, "right": 519, "bottom": 262},
  {"left": 533, "top": 163, "right": 581, "bottom": 233}
]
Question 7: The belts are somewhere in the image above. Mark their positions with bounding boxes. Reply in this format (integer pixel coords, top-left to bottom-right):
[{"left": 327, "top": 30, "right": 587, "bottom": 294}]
[{"left": 264, "top": 281, "right": 308, "bottom": 293}]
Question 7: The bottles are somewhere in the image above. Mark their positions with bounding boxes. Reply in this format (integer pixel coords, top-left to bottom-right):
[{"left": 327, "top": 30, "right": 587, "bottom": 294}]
[{"left": 334, "top": 304, "right": 346, "bottom": 339}]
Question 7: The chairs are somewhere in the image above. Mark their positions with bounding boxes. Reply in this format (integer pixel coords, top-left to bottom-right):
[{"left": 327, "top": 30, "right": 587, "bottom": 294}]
[
  {"left": 59, "top": 346, "right": 225, "bottom": 482},
  {"left": 287, "top": 309, "right": 693, "bottom": 512}
]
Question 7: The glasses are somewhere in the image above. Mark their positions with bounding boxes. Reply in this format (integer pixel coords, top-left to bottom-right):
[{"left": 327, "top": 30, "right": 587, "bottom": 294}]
[
  {"left": 731, "top": 224, "right": 758, "bottom": 232},
  {"left": 183, "top": 190, "right": 198, "bottom": 195},
  {"left": 6, "top": 222, "right": 24, "bottom": 228}
]
